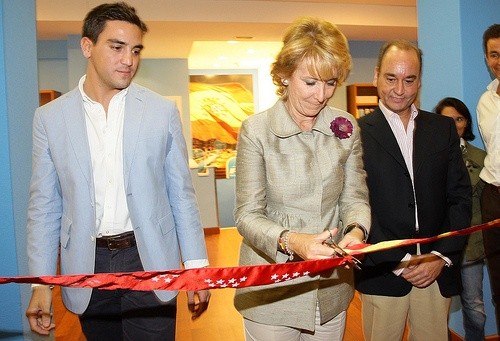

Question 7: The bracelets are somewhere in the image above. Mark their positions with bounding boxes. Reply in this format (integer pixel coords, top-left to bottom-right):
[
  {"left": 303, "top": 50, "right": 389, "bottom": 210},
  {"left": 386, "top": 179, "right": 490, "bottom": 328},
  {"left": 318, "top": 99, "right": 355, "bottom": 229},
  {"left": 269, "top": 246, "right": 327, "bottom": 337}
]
[
  {"left": 278, "top": 230, "right": 295, "bottom": 260},
  {"left": 344, "top": 223, "right": 367, "bottom": 240}
]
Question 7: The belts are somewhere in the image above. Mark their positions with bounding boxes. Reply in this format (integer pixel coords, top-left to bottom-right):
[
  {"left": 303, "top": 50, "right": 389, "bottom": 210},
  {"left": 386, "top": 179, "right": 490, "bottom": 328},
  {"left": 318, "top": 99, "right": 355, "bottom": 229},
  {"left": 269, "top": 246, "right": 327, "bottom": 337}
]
[
  {"left": 96, "top": 231, "right": 136, "bottom": 251},
  {"left": 415, "top": 230, "right": 419, "bottom": 240}
]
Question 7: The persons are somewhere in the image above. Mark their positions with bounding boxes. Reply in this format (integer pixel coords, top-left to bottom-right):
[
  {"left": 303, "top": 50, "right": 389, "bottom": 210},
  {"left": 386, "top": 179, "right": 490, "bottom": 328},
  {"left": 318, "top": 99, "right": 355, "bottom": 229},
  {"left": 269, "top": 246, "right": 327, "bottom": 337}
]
[
  {"left": 476, "top": 24, "right": 500, "bottom": 338},
  {"left": 25, "top": 3, "right": 211, "bottom": 341},
  {"left": 355, "top": 41, "right": 473, "bottom": 341},
  {"left": 234, "top": 16, "right": 371, "bottom": 341},
  {"left": 434, "top": 96, "right": 488, "bottom": 341}
]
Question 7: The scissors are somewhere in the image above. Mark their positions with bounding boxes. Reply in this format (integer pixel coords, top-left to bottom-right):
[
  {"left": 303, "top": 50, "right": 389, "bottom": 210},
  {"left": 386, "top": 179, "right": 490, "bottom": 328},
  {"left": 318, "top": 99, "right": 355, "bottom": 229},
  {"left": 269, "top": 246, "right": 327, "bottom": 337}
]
[{"left": 322, "top": 228, "right": 362, "bottom": 269}]
